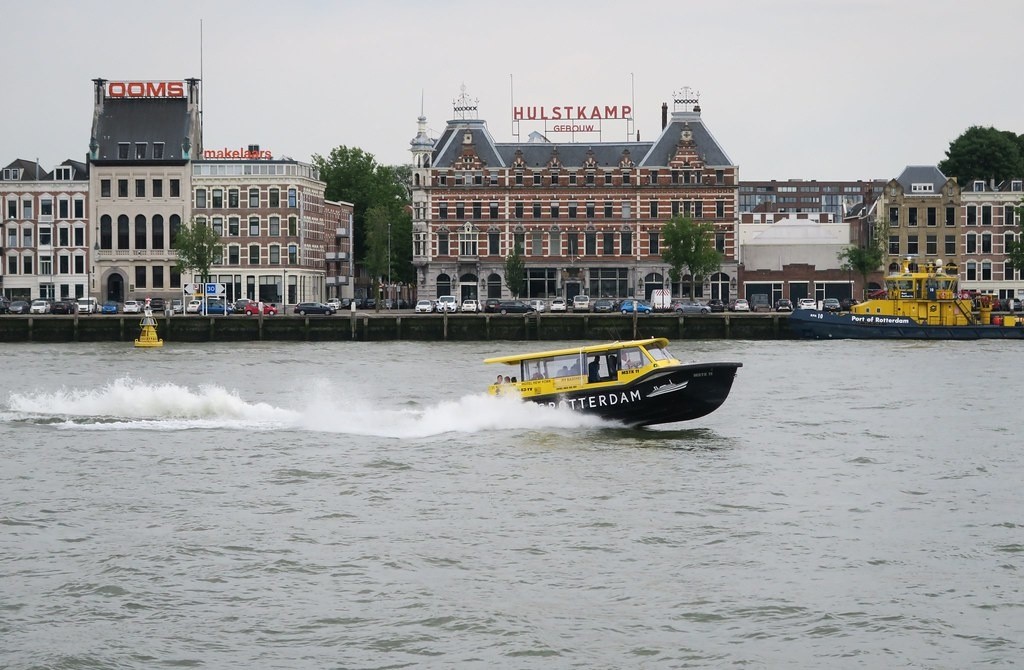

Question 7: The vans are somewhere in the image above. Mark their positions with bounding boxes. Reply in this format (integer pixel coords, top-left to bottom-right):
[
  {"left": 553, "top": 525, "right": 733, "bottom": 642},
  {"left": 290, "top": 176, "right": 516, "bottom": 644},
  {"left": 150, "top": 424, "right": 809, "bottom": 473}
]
[
  {"left": 572, "top": 294, "right": 590, "bottom": 311},
  {"left": 78, "top": 297, "right": 97, "bottom": 313}
]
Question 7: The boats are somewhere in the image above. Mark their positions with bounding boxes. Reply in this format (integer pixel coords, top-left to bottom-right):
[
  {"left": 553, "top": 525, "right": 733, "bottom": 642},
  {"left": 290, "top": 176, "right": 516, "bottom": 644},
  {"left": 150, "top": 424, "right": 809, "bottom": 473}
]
[
  {"left": 134, "top": 316, "right": 164, "bottom": 347},
  {"left": 788, "top": 257, "right": 1024, "bottom": 340},
  {"left": 482, "top": 335, "right": 743, "bottom": 430}
]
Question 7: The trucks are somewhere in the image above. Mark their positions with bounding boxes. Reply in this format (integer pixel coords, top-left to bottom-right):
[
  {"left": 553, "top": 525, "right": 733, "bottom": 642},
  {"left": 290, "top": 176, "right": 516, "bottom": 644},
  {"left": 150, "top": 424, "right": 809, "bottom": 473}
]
[{"left": 651, "top": 289, "right": 672, "bottom": 312}]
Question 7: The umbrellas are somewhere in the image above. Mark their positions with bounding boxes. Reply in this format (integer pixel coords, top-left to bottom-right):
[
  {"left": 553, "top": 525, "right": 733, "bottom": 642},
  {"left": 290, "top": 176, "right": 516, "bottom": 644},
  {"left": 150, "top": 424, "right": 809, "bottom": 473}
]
[{"left": 865, "top": 289, "right": 887, "bottom": 300}]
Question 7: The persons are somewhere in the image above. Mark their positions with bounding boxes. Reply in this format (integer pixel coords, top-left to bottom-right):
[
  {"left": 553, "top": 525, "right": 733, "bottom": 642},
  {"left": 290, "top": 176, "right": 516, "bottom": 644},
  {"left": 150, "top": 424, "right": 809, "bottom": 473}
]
[
  {"left": 494, "top": 374, "right": 518, "bottom": 385},
  {"left": 616, "top": 353, "right": 637, "bottom": 375},
  {"left": 556, "top": 355, "right": 601, "bottom": 383}
]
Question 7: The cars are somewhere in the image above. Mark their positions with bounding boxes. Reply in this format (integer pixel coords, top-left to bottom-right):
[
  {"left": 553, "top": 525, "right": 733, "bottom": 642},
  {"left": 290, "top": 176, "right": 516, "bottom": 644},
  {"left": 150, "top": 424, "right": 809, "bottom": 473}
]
[
  {"left": 594, "top": 298, "right": 750, "bottom": 314},
  {"left": 414, "top": 295, "right": 568, "bottom": 314},
  {"left": 775, "top": 297, "right": 859, "bottom": 312},
  {"left": 1005, "top": 299, "right": 1022, "bottom": 310},
  {"left": 0, "top": 294, "right": 277, "bottom": 315},
  {"left": 293, "top": 297, "right": 408, "bottom": 315}
]
